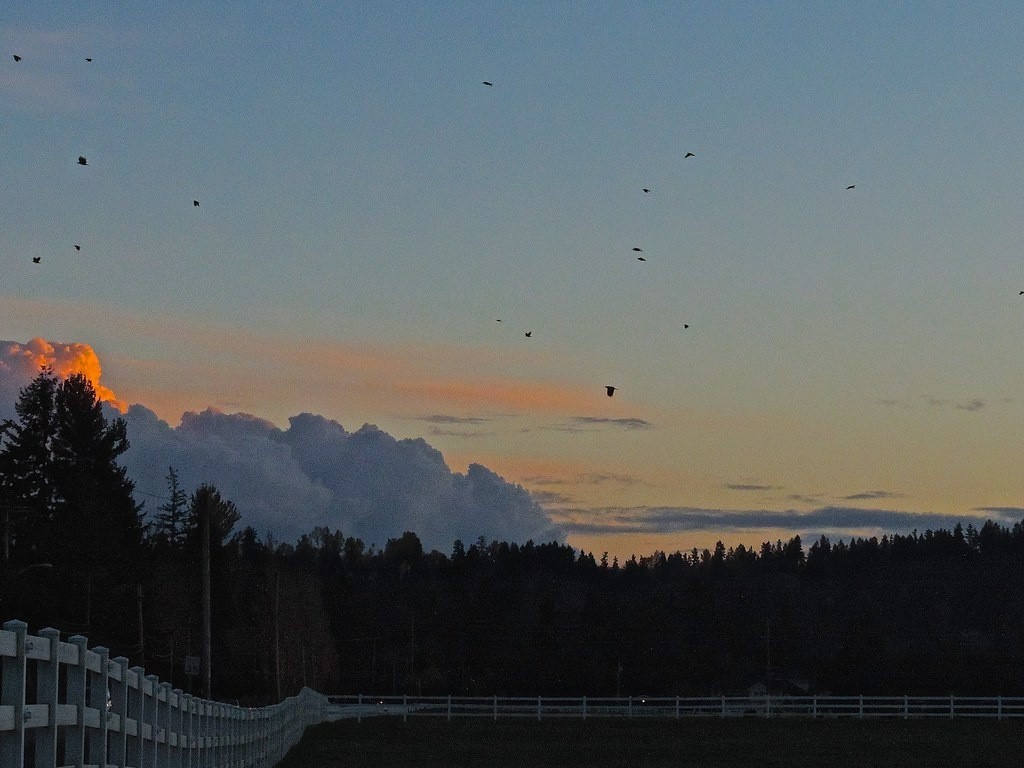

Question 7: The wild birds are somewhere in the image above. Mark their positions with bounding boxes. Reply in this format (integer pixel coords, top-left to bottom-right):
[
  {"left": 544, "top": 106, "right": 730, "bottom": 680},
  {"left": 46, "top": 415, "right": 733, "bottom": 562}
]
[
  {"left": 496, "top": 319, "right": 502, "bottom": 323},
  {"left": 845, "top": 184, "right": 857, "bottom": 191},
  {"left": 12, "top": 54, "right": 22, "bottom": 63},
  {"left": 631, "top": 244, "right": 644, "bottom": 253},
  {"left": 482, "top": 81, "right": 494, "bottom": 89},
  {"left": 193, "top": 199, "right": 200, "bottom": 207},
  {"left": 605, "top": 385, "right": 619, "bottom": 397},
  {"left": 74, "top": 243, "right": 81, "bottom": 251},
  {"left": 683, "top": 150, "right": 695, "bottom": 159},
  {"left": 1017, "top": 288, "right": 1023, "bottom": 298},
  {"left": 638, "top": 256, "right": 646, "bottom": 262},
  {"left": 84, "top": 58, "right": 93, "bottom": 62},
  {"left": 525, "top": 331, "right": 532, "bottom": 338},
  {"left": 683, "top": 323, "right": 689, "bottom": 329},
  {"left": 76, "top": 156, "right": 89, "bottom": 166},
  {"left": 32, "top": 256, "right": 41, "bottom": 264},
  {"left": 642, "top": 186, "right": 650, "bottom": 193}
]
